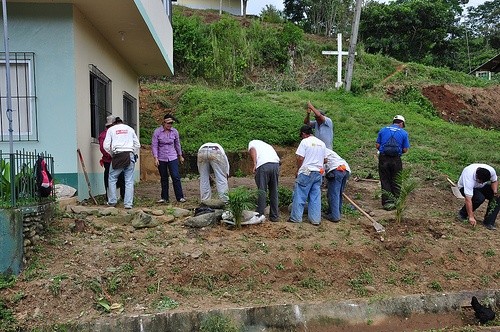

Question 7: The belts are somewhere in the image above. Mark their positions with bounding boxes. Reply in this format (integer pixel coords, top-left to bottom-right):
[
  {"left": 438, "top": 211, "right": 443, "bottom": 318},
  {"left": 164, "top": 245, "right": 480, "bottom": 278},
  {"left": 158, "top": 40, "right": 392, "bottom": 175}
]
[{"left": 202, "top": 146, "right": 218, "bottom": 149}]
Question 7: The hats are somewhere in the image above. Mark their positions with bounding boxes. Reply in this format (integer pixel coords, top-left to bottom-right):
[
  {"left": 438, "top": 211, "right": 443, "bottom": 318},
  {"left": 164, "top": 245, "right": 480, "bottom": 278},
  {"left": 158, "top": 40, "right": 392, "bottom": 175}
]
[
  {"left": 393, "top": 114, "right": 406, "bottom": 127},
  {"left": 105, "top": 115, "right": 115, "bottom": 126},
  {"left": 313, "top": 111, "right": 325, "bottom": 117},
  {"left": 299, "top": 126, "right": 312, "bottom": 137},
  {"left": 164, "top": 118, "right": 174, "bottom": 124}
]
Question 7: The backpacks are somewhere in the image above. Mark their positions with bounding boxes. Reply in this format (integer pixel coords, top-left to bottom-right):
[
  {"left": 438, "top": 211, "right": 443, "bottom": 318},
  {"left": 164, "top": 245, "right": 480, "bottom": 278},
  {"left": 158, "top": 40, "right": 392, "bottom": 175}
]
[{"left": 384, "top": 128, "right": 401, "bottom": 155}]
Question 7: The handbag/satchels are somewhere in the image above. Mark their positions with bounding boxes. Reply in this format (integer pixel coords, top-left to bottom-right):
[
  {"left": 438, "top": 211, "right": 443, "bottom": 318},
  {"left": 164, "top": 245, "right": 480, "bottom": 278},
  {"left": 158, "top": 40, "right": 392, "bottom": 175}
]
[{"left": 112, "top": 151, "right": 131, "bottom": 168}]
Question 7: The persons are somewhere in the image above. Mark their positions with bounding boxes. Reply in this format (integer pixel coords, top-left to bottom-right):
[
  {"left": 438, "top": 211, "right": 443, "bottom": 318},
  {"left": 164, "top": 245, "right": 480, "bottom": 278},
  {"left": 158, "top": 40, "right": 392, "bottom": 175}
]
[
  {"left": 324, "top": 148, "right": 352, "bottom": 222},
  {"left": 458, "top": 163, "right": 500, "bottom": 229},
  {"left": 248, "top": 140, "right": 280, "bottom": 221},
  {"left": 197, "top": 142, "right": 229, "bottom": 202},
  {"left": 287, "top": 126, "right": 328, "bottom": 225},
  {"left": 97, "top": 115, "right": 141, "bottom": 208},
  {"left": 375, "top": 115, "right": 409, "bottom": 206},
  {"left": 151, "top": 113, "right": 186, "bottom": 203},
  {"left": 304, "top": 101, "right": 333, "bottom": 151}
]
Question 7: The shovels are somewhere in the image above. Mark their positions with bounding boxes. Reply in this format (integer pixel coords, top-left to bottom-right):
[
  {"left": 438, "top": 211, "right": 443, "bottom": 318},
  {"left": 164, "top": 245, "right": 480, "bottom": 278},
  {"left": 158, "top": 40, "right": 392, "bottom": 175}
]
[
  {"left": 77, "top": 149, "right": 98, "bottom": 205},
  {"left": 341, "top": 192, "right": 386, "bottom": 233},
  {"left": 446, "top": 177, "right": 464, "bottom": 199}
]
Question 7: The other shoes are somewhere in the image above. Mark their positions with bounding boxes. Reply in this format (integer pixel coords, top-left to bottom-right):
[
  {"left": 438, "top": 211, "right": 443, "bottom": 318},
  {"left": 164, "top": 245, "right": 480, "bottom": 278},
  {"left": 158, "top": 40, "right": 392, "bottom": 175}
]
[
  {"left": 179, "top": 198, "right": 187, "bottom": 203},
  {"left": 456, "top": 215, "right": 468, "bottom": 222},
  {"left": 483, "top": 222, "right": 496, "bottom": 230},
  {"left": 107, "top": 202, "right": 116, "bottom": 207},
  {"left": 156, "top": 198, "right": 169, "bottom": 203}
]
